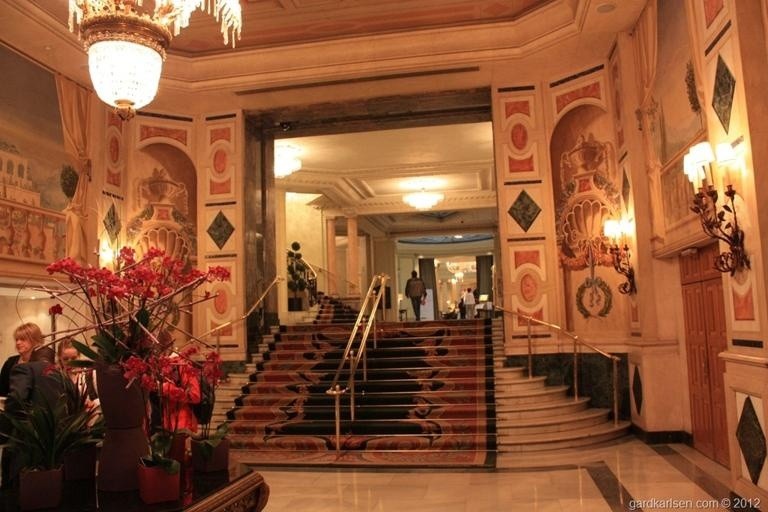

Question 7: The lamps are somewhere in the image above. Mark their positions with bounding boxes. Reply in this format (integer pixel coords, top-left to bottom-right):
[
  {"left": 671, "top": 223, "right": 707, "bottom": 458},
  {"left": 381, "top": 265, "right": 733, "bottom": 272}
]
[
  {"left": 683, "top": 140, "right": 752, "bottom": 276},
  {"left": 68, "top": 0, "right": 243, "bottom": 122},
  {"left": 401, "top": 188, "right": 444, "bottom": 212},
  {"left": 273, "top": 139, "right": 304, "bottom": 180},
  {"left": 603, "top": 219, "right": 637, "bottom": 297}
]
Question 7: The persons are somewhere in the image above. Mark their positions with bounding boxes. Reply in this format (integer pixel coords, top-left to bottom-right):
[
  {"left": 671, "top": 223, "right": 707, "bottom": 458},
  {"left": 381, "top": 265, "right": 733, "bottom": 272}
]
[
  {"left": 459, "top": 287, "right": 479, "bottom": 319},
  {"left": 146, "top": 329, "right": 201, "bottom": 433},
  {"left": 0, "top": 322, "right": 105, "bottom": 443},
  {"left": 406, "top": 271, "right": 427, "bottom": 321}
]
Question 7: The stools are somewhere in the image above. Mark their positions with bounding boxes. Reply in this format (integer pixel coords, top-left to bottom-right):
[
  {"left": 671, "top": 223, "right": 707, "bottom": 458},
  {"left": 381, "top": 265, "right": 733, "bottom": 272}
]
[{"left": 399, "top": 309, "right": 407, "bottom": 322}]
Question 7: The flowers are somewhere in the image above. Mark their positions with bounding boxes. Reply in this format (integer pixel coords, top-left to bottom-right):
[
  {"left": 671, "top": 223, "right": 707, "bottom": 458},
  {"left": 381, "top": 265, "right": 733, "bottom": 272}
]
[{"left": 42, "top": 245, "right": 230, "bottom": 435}]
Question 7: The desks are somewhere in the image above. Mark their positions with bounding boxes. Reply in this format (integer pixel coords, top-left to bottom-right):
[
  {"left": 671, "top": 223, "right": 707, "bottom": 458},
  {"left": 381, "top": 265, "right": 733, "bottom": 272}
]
[{"left": 474, "top": 302, "right": 493, "bottom": 320}]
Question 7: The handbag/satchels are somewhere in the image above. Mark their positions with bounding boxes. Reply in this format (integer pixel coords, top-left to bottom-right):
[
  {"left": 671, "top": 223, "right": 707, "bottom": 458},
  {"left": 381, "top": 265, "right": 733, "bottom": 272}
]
[{"left": 189, "top": 379, "right": 214, "bottom": 423}]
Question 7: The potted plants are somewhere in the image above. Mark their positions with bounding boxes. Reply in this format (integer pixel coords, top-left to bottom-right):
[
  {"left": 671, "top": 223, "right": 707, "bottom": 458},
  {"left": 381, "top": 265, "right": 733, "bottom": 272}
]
[
  {"left": 65, "top": 302, "right": 149, "bottom": 428},
  {"left": 2, "top": 372, "right": 107, "bottom": 511},
  {"left": 193, "top": 423, "right": 231, "bottom": 475},
  {"left": 148, "top": 419, "right": 198, "bottom": 465},
  {"left": 138, "top": 446, "right": 184, "bottom": 505}
]
